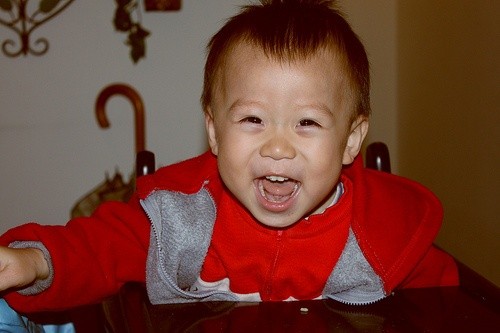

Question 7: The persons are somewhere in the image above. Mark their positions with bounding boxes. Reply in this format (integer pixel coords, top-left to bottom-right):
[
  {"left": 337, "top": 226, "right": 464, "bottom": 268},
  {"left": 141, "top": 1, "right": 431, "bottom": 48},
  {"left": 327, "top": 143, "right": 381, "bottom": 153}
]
[{"left": 0, "top": 1, "right": 461, "bottom": 327}]
